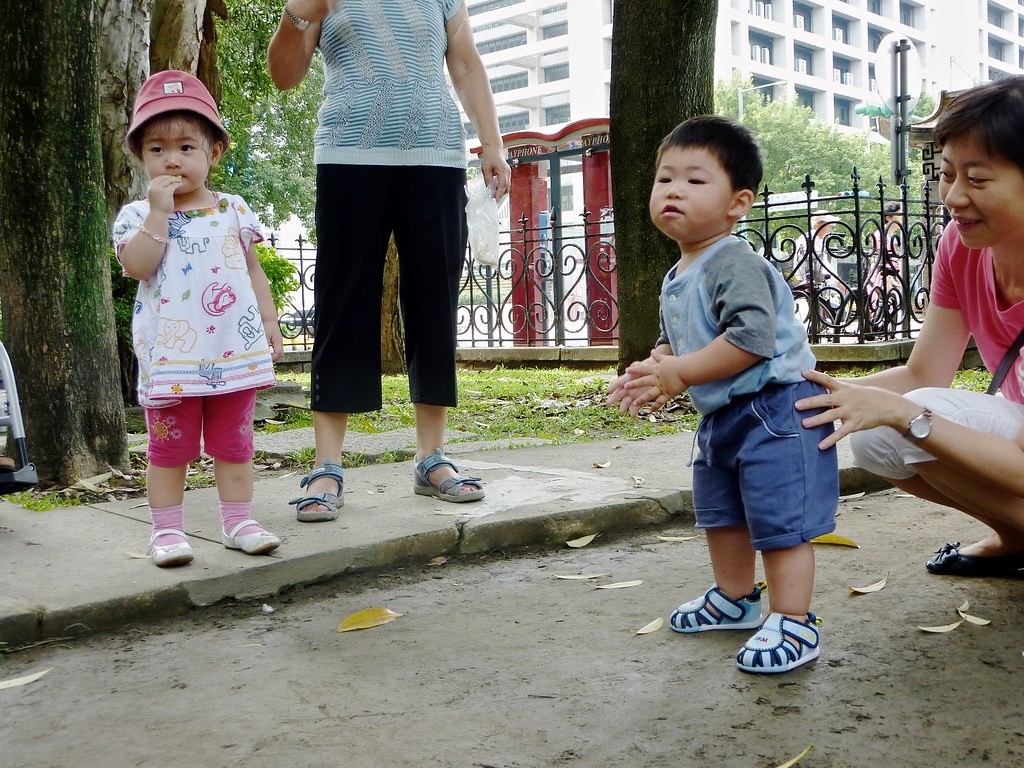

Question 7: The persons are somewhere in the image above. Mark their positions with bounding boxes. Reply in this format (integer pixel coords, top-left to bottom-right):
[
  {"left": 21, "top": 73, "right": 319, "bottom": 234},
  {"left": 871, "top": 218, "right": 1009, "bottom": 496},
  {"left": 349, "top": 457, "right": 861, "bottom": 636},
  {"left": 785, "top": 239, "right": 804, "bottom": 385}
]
[
  {"left": 794, "top": 74, "right": 1024, "bottom": 577},
  {"left": 109, "top": 65, "right": 285, "bottom": 566},
  {"left": 269, "top": 0, "right": 515, "bottom": 523},
  {"left": 602, "top": 116, "right": 841, "bottom": 687}
]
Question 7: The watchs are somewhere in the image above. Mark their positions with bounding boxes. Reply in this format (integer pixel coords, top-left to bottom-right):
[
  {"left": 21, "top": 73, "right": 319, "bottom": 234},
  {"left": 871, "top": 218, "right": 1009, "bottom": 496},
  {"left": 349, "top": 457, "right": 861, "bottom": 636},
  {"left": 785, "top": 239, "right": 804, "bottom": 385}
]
[
  {"left": 903, "top": 408, "right": 934, "bottom": 442},
  {"left": 284, "top": 5, "right": 310, "bottom": 31}
]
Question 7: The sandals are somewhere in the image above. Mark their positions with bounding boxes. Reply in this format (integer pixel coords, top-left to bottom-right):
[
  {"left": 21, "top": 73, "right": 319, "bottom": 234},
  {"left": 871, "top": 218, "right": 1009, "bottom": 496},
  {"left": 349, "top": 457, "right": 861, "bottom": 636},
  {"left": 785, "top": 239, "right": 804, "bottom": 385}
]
[
  {"left": 736, "top": 612, "right": 821, "bottom": 672},
  {"left": 414, "top": 453, "right": 487, "bottom": 504},
  {"left": 669, "top": 583, "right": 762, "bottom": 632},
  {"left": 295, "top": 462, "right": 346, "bottom": 520}
]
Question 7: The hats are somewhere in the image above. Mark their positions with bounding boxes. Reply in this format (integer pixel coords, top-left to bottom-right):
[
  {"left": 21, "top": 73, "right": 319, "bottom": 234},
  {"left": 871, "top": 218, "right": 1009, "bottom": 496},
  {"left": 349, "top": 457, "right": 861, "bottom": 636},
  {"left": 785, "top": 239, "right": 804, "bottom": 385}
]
[{"left": 126, "top": 70, "right": 231, "bottom": 158}]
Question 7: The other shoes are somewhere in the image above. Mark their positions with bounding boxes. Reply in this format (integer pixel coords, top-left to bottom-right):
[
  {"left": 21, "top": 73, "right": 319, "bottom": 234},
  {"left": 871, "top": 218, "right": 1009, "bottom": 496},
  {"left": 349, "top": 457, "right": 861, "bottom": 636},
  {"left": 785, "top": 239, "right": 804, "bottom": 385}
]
[
  {"left": 149, "top": 527, "right": 193, "bottom": 568},
  {"left": 221, "top": 519, "right": 279, "bottom": 554},
  {"left": 927, "top": 541, "right": 1023, "bottom": 575}
]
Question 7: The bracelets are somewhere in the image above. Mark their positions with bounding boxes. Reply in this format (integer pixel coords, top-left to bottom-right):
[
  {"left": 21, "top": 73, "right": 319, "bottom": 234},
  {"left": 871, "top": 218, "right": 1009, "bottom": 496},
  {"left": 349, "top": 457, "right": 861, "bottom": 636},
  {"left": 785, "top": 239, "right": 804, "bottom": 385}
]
[{"left": 138, "top": 224, "right": 170, "bottom": 247}]
